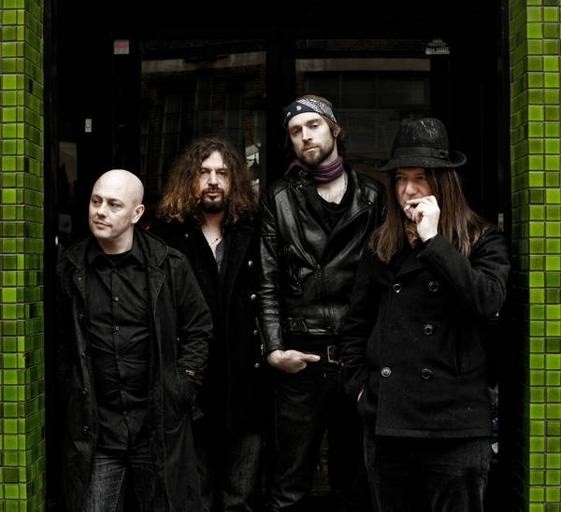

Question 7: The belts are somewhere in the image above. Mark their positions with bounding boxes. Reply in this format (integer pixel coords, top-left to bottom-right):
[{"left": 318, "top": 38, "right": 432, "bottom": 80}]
[{"left": 324, "top": 344, "right": 343, "bottom": 365}]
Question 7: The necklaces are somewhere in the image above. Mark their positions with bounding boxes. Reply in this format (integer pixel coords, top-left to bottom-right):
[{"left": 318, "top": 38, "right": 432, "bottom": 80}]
[{"left": 209, "top": 236, "right": 222, "bottom": 246}]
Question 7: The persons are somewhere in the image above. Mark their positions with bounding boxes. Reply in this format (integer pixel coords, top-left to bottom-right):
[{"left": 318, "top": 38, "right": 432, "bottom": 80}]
[
  {"left": 333, "top": 118, "right": 513, "bottom": 512},
  {"left": 44, "top": 167, "right": 214, "bottom": 512},
  {"left": 150, "top": 135, "right": 260, "bottom": 509},
  {"left": 242, "top": 95, "right": 386, "bottom": 512}
]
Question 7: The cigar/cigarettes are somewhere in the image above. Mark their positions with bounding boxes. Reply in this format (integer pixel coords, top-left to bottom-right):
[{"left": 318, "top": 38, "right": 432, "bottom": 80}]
[{"left": 403, "top": 204, "right": 411, "bottom": 211}]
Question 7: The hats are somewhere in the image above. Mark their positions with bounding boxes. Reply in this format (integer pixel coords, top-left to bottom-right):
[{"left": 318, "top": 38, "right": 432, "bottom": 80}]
[{"left": 375, "top": 117, "right": 466, "bottom": 174}]
